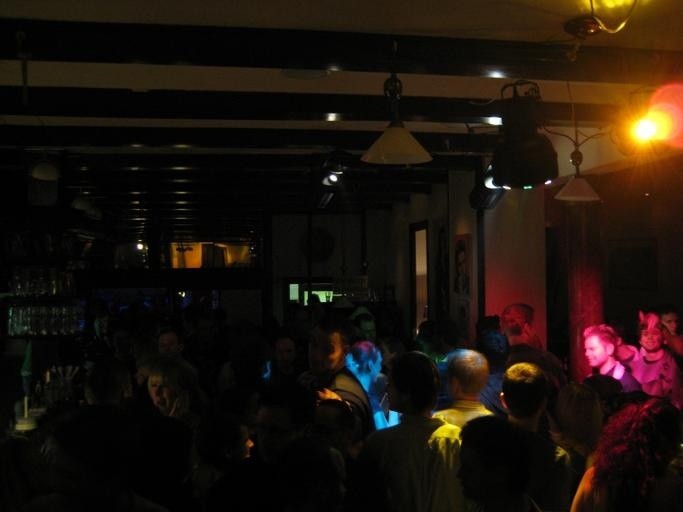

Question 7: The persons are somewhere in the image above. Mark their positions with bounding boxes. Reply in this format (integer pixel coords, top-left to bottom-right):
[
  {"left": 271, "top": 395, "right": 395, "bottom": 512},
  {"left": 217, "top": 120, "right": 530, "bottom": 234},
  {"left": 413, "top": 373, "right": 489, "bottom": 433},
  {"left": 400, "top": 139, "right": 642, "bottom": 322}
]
[
  {"left": 60, "top": 272, "right": 77, "bottom": 296},
  {"left": 1, "top": 296, "right": 683, "bottom": 512}
]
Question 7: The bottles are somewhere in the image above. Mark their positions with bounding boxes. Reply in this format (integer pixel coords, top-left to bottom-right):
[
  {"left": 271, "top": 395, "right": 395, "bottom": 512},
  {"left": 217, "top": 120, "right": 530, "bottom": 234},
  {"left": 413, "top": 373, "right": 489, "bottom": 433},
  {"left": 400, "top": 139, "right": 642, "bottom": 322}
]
[{"left": 6, "top": 263, "right": 81, "bottom": 338}]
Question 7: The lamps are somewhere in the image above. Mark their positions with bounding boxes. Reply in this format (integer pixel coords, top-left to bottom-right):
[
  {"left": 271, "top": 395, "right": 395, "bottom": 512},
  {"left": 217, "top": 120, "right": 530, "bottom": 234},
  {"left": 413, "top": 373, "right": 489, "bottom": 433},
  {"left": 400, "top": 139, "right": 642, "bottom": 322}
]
[
  {"left": 490, "top": 82, "right": 558, "bottom": 190},
  {"left": 360, "top": 77, "right": 435, "bottom": 168},
  {"left": 543, "top": 73, "right": 609, "bottom": 203},
  {"left": 462, "top": 115, "right": 505, "bottom": 217}
]
